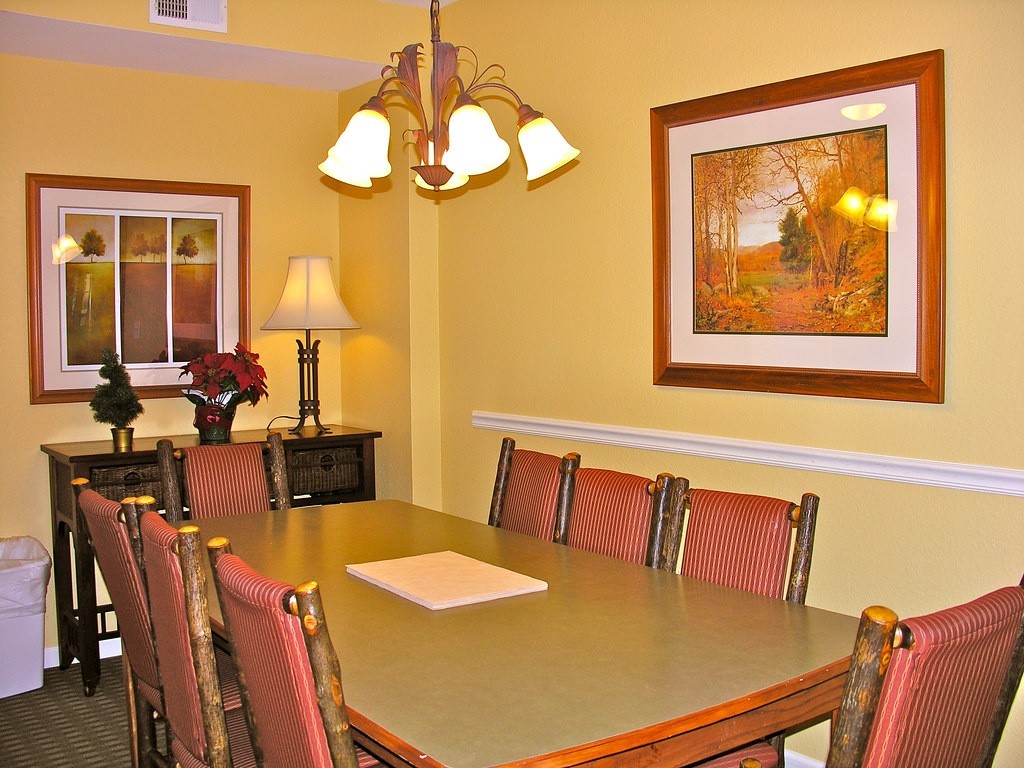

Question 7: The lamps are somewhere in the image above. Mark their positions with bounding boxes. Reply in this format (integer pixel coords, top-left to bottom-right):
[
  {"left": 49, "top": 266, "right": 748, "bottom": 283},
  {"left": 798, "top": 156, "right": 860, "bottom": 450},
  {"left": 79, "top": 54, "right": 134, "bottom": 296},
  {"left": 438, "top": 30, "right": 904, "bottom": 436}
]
[
  {"left": 260, "top": 255, "right": 364, "bottom": 435},
  {"left": 317, "top": 1, "right": 581, "bottom": 193}
]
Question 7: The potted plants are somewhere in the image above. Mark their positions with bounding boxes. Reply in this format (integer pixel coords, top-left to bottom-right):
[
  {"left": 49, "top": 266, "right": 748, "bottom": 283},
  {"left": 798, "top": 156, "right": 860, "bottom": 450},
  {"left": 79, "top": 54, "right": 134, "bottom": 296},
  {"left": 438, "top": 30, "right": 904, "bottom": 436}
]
[{"left": 89, "top": 347, "right": 145, "bottom": 446}]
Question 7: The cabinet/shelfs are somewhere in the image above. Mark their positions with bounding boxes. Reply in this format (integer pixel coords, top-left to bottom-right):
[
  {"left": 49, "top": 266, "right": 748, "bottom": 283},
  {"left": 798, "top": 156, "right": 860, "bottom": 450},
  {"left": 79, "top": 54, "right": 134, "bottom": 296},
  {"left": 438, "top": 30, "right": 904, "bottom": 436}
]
[{"left": 39, "top": 423, "right": 383, "bottom": 698}]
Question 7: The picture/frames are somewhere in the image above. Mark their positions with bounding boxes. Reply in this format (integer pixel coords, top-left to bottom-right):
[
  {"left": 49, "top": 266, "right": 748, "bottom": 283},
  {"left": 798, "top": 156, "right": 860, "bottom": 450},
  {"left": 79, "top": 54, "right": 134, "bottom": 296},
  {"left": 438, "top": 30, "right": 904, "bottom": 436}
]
[
  {"left": 649, "top": 49, "right": 948, "bottom": 406},
  {"left": 25, "top": 172, "right": 253, "bottom": 404}
]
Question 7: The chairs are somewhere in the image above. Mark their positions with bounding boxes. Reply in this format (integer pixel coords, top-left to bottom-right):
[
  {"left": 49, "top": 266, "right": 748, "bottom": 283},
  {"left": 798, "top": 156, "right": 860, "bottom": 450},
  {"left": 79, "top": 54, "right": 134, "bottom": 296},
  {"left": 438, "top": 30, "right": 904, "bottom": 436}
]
[
  {"left": 661, "top": 477, "right": 820, "bottom": 607},
  {"left": 687, "top": 575, "right": 1024, "bottom": 768},
  {"left": 68, "top": 478, "right": 395, "bottom": 768},
  {"left": 488, "top": 438, "right": 581, "bottom": 543},
  {"left": 552, "top": 455, "right": 674, "bottom": 568},
  {"left": 156, "top": 432, "right": 291, "bottom": 524}
]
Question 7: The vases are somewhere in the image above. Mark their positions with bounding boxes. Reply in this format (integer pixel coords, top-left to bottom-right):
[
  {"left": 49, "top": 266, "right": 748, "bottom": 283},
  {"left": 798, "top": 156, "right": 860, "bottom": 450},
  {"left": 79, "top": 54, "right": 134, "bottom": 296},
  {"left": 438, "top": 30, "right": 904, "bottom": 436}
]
[{"left": 192, "top": 405, "right": 235, "bottom": 444}]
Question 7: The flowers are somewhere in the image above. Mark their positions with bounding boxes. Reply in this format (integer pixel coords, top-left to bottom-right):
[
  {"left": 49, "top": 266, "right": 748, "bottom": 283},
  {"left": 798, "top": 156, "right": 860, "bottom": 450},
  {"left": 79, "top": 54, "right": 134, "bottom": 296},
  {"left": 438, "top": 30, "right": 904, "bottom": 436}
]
[{"left": 175, "top": 342, "right": 269, "bottom": 410}]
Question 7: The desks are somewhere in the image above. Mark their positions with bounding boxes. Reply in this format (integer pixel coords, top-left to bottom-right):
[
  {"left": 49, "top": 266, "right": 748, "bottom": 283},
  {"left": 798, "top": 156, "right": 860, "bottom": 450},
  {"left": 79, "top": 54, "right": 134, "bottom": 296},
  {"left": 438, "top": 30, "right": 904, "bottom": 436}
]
[{"left": 156, "top": 498, "right": 862, "bottom": 768}]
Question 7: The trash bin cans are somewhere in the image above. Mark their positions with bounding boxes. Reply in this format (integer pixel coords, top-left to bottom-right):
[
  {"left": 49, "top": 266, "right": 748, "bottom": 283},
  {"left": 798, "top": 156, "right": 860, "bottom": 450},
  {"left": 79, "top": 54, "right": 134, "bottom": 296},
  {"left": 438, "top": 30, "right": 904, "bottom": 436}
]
[{"left": 0, "top": 535, "right": 52, "bottom": 699}]
